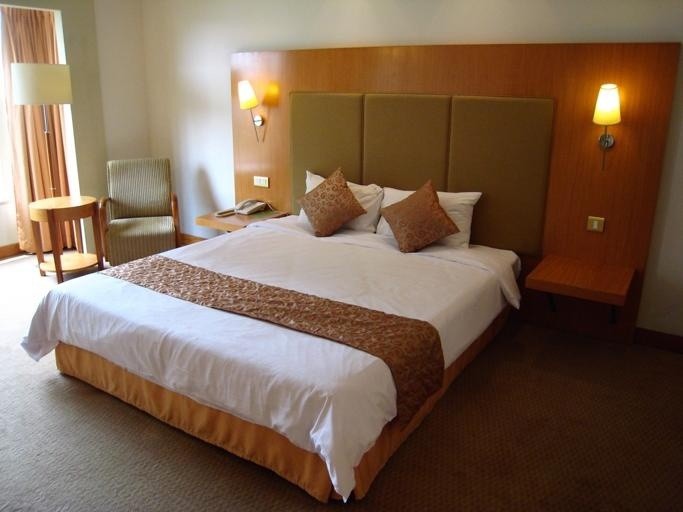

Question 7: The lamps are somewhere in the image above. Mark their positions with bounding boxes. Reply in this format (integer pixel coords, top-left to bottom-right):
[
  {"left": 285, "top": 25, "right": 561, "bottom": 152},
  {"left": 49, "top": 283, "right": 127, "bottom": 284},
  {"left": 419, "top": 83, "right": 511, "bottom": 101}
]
[
  {"left": 10, "top": 62, "right": 73, "bottom": 197},
  {"left": 238, "top": 80, "right": 264, "bottom": 127},
  {"left": 592, "top": 83, "right": 622, "bottom": 149}
]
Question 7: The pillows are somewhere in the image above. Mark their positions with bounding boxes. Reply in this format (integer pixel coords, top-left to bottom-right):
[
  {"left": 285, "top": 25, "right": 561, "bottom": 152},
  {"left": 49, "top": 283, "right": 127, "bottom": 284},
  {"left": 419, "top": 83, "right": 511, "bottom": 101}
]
[{"left": 294, "top": 167, "right": 482, "bottom": 253}]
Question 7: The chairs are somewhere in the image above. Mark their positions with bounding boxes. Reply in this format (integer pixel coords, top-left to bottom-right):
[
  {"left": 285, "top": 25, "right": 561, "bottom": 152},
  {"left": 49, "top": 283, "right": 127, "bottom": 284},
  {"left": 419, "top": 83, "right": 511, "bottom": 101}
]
[{"left": 99, "top": 157, "right": 181, "bottom": 266}]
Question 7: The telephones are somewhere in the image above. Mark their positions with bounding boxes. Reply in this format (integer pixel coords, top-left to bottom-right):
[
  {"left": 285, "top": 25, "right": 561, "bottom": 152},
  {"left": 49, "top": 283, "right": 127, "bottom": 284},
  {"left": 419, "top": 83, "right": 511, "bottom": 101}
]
[{"left": 234, "top": 199, "right": 266, "bottom": 215}]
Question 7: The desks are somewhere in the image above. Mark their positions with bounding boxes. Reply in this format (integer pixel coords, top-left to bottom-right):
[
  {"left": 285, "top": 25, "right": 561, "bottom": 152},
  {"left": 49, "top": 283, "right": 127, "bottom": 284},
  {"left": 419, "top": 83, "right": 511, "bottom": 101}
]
[{"left": 28, "top": 195, "right": 104, "bottom": 284}]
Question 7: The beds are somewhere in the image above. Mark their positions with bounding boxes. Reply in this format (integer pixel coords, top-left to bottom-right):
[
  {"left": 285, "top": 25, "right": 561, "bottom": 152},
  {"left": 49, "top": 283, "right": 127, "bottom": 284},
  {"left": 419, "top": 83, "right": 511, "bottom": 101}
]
[{"left": 55, "top": 91, "right": 555, "bottom": 506}]
